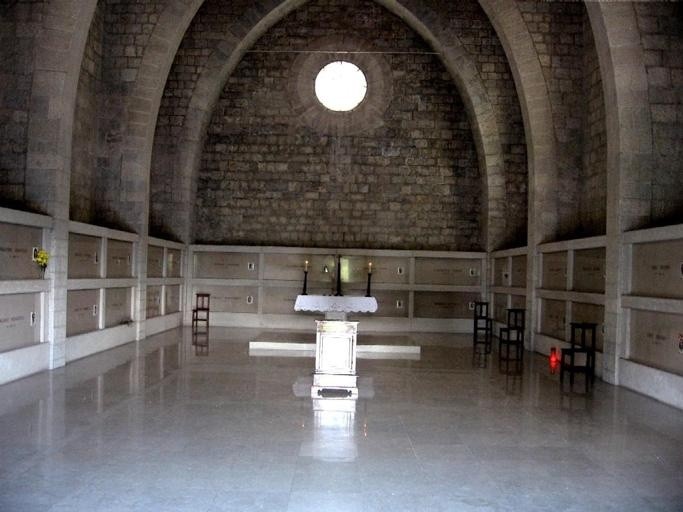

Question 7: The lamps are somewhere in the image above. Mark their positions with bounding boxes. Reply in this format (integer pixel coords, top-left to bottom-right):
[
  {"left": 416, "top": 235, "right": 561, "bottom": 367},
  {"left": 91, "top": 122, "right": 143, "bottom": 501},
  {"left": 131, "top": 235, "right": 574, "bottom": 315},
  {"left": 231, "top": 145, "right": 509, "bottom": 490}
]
[{"left": 547, "top": 345, "right": 558, "bottom": 377}]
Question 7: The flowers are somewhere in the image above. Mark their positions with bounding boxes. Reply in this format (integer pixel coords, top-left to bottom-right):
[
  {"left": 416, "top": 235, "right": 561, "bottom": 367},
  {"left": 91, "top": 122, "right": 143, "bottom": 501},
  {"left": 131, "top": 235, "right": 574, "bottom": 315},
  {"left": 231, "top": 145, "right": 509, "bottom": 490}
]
[{"left": 33, "top": 250, "right": 48, "bottom": 273}]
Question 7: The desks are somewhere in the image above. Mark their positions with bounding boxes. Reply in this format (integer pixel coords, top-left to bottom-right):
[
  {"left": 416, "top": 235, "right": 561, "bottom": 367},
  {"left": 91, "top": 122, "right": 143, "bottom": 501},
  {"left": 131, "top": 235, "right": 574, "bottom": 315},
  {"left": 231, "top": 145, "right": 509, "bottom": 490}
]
[
  {"left": 293, "top": 294, "right": 379, "bottom": 322},
  {"left": 291, "top": 371, "right": 375, "bottom": 398}
]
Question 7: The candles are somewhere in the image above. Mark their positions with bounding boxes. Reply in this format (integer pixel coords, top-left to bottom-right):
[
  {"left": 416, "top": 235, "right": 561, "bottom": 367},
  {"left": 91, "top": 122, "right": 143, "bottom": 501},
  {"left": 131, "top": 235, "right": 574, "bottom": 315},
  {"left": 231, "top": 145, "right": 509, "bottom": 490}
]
[
  {"left": 304, "top": 259, "right": 308, "bottom": 272},
  {"left": 368, "top": 261, "right": 372, "bottom": 272}
]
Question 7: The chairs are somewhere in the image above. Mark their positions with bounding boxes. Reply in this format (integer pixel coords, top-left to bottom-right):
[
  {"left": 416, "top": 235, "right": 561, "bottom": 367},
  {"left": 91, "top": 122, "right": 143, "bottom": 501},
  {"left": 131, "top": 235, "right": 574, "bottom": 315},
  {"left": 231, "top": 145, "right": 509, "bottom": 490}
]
[
  {"left": 472, "top": 299, "right": 494, "bottom": 345},
  {"left": 498, "top": 307, "right": 527, "bottom": 359},
  {"left": 472, "top": 345, "right": 492, "bottom": 370},
  {"left": 560, "top": 321, "right": 598, "bottom": 386},
  {"left": 498, "top": 360, "right": 523, "bottom": 397},
  {"left": 190, "top": 292, "right": 210, "bottom": 328},
  {"left": 191, "top": 328, "right": 209, "bottom": 357},
  {"left": 559, "top": 387, "right": 595, "bottom": 425}
]
